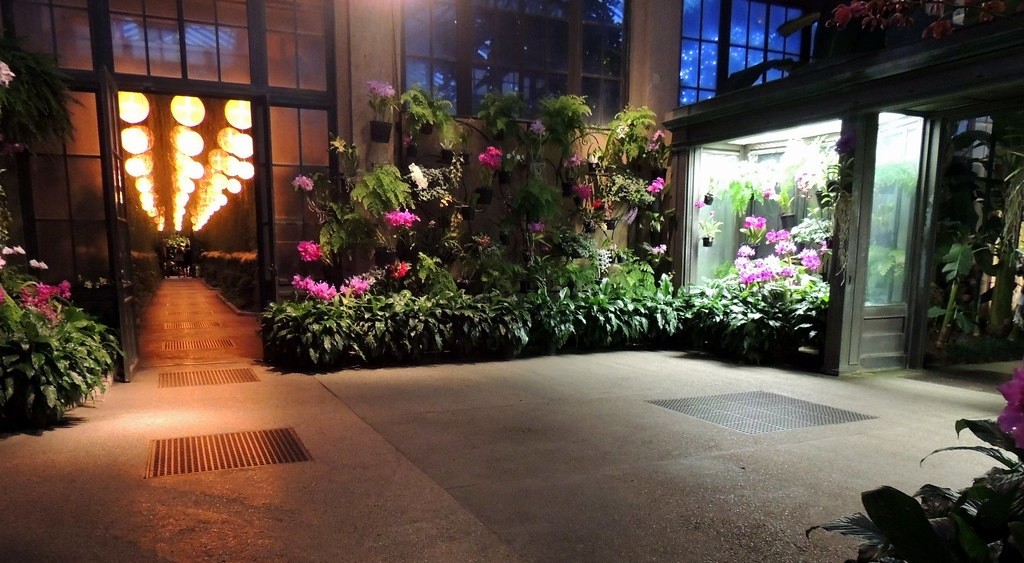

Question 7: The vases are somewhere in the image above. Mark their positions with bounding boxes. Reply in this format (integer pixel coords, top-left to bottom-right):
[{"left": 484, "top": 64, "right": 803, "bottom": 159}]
[
  {"left": 582, "top": 222, "right": 596, "bottom": 232},
  {"left": 437, "top": 216, "right": 451, "bottom": 229},
  {"left": 649, "top": 166, "right": 667, "bottom": 180},
  {"left": 476, "top": 187, "right": 493, "bottom": 207},
  {"left": 605, "top": 219, "right": 616, "bottom": 229},
  {"left": 406, "top": 146, "right": 417, "bottom": 157},
  {"left": 520, "top": 275, "right": 538, "bottom": 292},
  {"left": 561, "top": 183, "right": 572, "bottom": 197},
  {"left": 369, "top": 119, "right": 393, "bottom": 143},
  {"left": 703, "top": 194, "right": 714, "bottom": 206},
  {"left": 457, "top": 281, "right": 478, "bottom": 295},
  {"left": 499, "top": 230, "right": 511, "bottom": 245}
]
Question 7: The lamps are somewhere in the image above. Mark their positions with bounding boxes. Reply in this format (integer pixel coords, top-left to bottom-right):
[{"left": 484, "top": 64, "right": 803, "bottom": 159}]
[{"left": 118, "top": 91, "right": 255, "bottom": 232}]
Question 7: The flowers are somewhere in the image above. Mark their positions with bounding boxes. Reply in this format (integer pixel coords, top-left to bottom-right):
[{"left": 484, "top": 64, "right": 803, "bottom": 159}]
[
  {"left": 522, "top": 220, "right": 548, "bottom": 269},
  {"left": 0, "top": 34, "right": 74, "bottom": 330},
  {"left": 289, "top": 135, "right": 492, "bottom": 302},
  {"left": 502, "top": 188, "right": 514, "bottom": 234},
  {"left": 694, "top": 124, "right": 858, "bottom": 287},
  {"left": 562, "top": 154, "right": 582, "bottom": 185},
  {"left": 402, "top": 131, "right": 424, "bottom": 148},
  {"left": 824, "top": 0, "right": 1024, "bottom": 39},
  {"left": 575, "top": 103, "right": 676, "bottom": 232},
  {"left": 478, "top": 145, "right": 503, "bottom": 188},
  {"left": 804, "top": 367, "right": 1024, "bottom": 563},
  {"left": 366, "top": 79, "right": 401, "bottom": 122}
]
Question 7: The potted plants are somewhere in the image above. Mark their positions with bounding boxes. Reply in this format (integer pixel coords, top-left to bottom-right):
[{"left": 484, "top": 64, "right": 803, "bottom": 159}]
[
  {"left": 343, "top": 159, "right": 416, "bottom": 268},
  {"left": 586, "top": 147, "right": 603, "bottom": 172},
  {"left": 439, "top": 139, "right": 455, "bottom": 161},
  {"left": 255, "top": 272, "right": 820, "bottom": 376},
  {"left": 478, "top": 86, "right": 531, "bottom": 141},
  {"left": 400, "top": 83, "right": 454, "bottom": 135},
  {"left": 778, "top": 183, "right": 796, "bottom": 232},
  {"left": 815, "top": 184, "right": 832, "bottom": 209},
  {"left": 697, "top": 215, "right": 723, "bottom": 248},
  {"left": 823, "top": 161, "right": 843, "bottom": 193},
  {"left": 0, "top": 306, "right": 127, "bottom": 429},
  {"left": 515, "top": 177, "right": 565, "bottom": 224}
]
